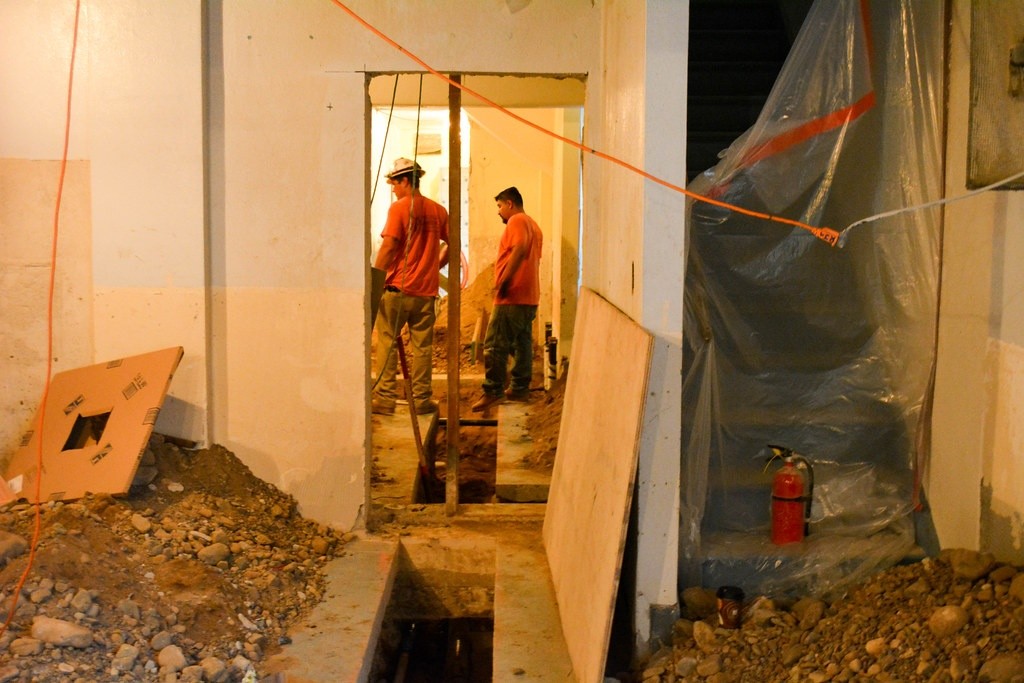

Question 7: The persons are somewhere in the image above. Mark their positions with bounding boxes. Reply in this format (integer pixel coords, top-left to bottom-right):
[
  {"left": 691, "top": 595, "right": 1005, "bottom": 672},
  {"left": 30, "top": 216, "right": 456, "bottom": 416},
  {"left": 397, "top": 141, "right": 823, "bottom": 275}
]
[
  {"left": 371, "top": 157, "right": 451, "bottom": 415},
  {"left": 471, "top": 186, "right": 543, "bottom": 413}
]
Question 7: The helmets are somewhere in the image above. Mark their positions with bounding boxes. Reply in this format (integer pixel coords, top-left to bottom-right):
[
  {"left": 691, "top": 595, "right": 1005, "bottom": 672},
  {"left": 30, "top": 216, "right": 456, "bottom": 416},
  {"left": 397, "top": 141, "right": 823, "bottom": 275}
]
[{"left": 384, "top": 157, "right": 426, "bottom": 184}]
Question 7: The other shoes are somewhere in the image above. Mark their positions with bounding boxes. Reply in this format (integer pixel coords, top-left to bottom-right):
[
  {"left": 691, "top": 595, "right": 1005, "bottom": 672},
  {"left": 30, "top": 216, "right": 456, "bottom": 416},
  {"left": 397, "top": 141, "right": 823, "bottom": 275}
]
[
  {"left": 416, "top": 400, "right": 438, "bottom": 415},
  {"left": 371, "top": 398, "right": 395, "bottom": 413},
  {"left": 472, "top": 390, "right": 506, "bottom": 412},
  {"left": 504, "top": 388, "right": 529, "bottom": 402}
]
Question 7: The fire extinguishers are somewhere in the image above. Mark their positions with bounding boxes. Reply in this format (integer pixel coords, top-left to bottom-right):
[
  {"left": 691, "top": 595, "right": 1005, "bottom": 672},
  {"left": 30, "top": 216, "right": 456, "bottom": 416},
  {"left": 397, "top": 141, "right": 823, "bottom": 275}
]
[{"left": 761, "top": 442, "right": 814, "bottom": 547}]
